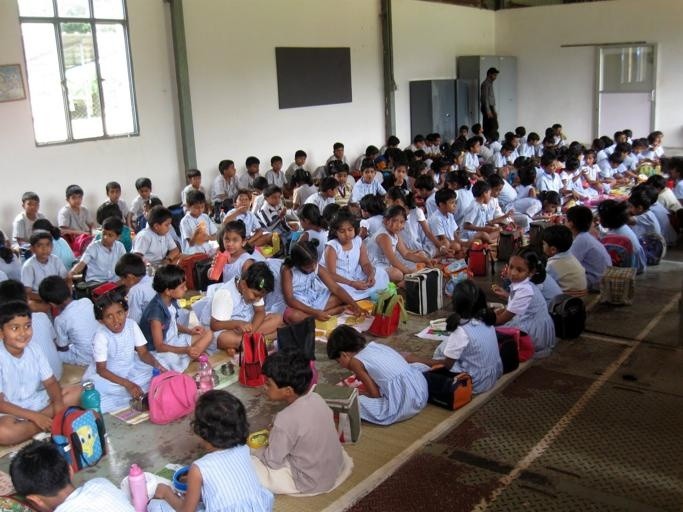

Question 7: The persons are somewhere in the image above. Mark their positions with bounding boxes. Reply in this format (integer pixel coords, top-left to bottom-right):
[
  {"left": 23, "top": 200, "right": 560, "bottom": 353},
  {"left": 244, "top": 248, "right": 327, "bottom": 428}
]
[
  {"left": 137, "top": 389, "right": 276, "bottom": 512},
  {"left": 326, "top": 324, "right": 428, "bottom": 424},
  {"left": 9, "top": 430, "right": 143, "bottom": 512},
  {"left": 1, "top": 301, "right": 85, "bottom": 447},
  {"left": 250, "top": 350, "right": 357, "bottom": 496},
  {"left": 0, "top": 123, "right": 683, "bottom": 413},
  {"left": 480, "top": 66, "right": 501, "bottom": 133}
]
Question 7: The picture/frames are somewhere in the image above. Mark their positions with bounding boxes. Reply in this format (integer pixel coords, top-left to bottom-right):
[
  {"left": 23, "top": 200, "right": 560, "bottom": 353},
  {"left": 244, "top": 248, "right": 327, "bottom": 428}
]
[{"left": 0, "top": 64, "right": 27, "bottom": 103}]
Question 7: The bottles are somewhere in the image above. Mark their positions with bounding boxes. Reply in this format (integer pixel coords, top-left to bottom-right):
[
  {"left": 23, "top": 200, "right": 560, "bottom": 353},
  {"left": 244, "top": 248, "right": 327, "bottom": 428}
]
[
  {"left": 79, "top": 382, "right": 106, "bottom": 437},
  {"left": 469, "top": 240, "right": 491, "bottom": 276},
  {"left": 498, "top": 230, "right": 513, "bottom": 262},
  {"left": 195, "top": 354, "right": 216, "bottom": 394},
  {"left": 555, "top": 206, "right": 563, "bottom": 224},
  {"left": 149, "top": 369, "right": 162, "bottom": 381},
  {"left": 219, "top": 207, "right": 226, "bottom": 224},
  {"left": 387, "top": 282, "right": 398, "bottom": 296},
  {"left": 145, "top": 262, "right": 154, "bottom": 277},
  {"left": 128, "top": 462, "right": 151, "bottom": 511},
  {"left": 197, "top": 218, "right": 206, "bottom": 240},
  {"left": 209, "top": 250, "right": 230, "bottom": 280},
  {"left": 270, "top": 230, "right": 281, "bottom": 254}
]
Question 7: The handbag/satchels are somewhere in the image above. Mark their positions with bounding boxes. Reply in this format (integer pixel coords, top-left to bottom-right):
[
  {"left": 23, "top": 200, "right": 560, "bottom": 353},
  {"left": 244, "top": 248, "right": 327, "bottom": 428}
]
[
  {"left": 424, "top": 365, "right": 472, "bottom": 411},
  {"left": 309, "top": 383, "right": 361, "bottom": 443},
  {"left": 148, "top": 371, "right": 197, "bottom": 424},
  {"left": 496, "top": 328, "right": 534, "bottom": 373}
]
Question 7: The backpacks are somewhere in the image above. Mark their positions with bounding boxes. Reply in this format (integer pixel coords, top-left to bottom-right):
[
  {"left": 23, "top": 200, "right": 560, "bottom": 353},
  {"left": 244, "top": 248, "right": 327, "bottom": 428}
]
[
  {"left": 639, "top": 233, "right": 666, "bottom": 264},
  {"left": 497, "top": 222, "right": 521, "bottom": 261},
  {"left": 239, "top": 332, "right": 268, "bottom": 387},
  {"left": 74, "top": 280, "right": 118, "bottom": 302},
  {"left": 549, "top": 295, "right": 586, "bottom": 339},
  {"left": 51, "top": 406, "right": 108, "bottom": 472},
  {"left": 444, "top": 243, "right": 494, "bottom": 297},
  {"left": 368, "top": 267, "right": 443, "bottom": 337},
  {"left": 181, "top": 253, "right": 222, "bottom": 291},
  {"left": 599, "top": 236, "right": 636, "bottom": 305}
]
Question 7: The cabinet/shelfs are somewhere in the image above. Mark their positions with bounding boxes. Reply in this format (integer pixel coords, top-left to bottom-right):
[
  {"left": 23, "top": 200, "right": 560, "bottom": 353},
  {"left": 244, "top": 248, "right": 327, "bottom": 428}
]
[
  {"left": 409, "top": 79, "right": 469, "bottom": 145},
  {"left": 458, "top": 55, "right": 518, "bottom": 139}
]
